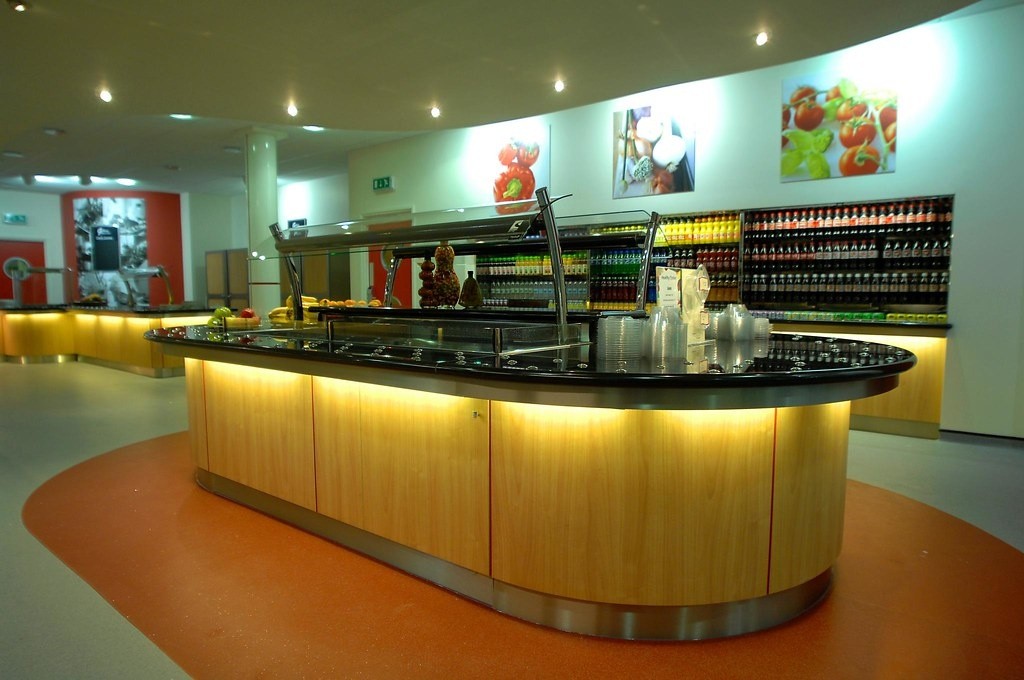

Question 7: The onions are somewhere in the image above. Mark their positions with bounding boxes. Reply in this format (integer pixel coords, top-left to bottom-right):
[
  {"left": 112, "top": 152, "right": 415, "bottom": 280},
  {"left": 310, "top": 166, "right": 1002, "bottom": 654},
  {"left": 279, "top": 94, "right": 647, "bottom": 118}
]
[{"left": 621, "top": 116, "right": 685, "bottom": 194}]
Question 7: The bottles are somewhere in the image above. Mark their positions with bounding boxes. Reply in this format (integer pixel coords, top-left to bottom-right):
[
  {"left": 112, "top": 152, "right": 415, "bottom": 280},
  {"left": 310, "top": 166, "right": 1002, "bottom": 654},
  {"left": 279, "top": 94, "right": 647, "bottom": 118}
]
[
  {"left": 647, "top": 214, "right": 739, "bottom": 311},
  {"left": 561, "top": 249, "right": 641, "bottom": 309},
  {"left": 476, "top": 255, "right": 555, "bottom": 309},
  {"left": 742, "top": 198, "right": 951, "bottom": 305}
]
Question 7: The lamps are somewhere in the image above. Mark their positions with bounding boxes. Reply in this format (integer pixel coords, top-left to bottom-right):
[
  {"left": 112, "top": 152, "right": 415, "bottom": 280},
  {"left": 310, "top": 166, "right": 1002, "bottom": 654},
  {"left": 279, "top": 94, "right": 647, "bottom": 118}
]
[{"left": 752, "top": 32, "right": 768, "bottom": 46}]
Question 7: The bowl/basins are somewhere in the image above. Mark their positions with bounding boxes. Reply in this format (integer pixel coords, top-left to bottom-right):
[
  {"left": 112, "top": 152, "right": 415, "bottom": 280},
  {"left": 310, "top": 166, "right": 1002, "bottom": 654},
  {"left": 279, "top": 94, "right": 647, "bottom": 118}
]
[
  {"left": 717, "top": 304, "right": 754, "bottom": 342},
  {"left": 645, "top": 305, "right": 688, "bottom": 359}
]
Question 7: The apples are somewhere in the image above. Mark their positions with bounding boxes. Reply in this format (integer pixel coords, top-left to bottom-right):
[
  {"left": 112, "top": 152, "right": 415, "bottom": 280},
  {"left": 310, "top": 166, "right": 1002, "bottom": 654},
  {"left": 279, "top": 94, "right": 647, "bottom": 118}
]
[{"left": 208, "top": 307, "right": 255, "bottom": 327}]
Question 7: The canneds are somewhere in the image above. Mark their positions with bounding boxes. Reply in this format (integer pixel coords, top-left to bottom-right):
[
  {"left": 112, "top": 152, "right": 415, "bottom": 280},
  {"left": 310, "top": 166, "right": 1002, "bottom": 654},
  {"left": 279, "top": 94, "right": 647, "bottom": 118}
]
[{"left": 748, "top": 309, "right": 948, "bottom": 324}]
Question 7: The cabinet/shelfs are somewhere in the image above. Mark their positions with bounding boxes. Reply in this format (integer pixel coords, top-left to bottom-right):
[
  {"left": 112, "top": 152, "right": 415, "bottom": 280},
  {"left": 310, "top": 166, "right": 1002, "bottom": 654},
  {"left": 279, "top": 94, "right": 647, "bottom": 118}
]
[
  {"left": 205, "top": 245, "right": 350, "bottom": 315},
  {"left": 464, "top": 194, "right": 955, "bottom": 328}
]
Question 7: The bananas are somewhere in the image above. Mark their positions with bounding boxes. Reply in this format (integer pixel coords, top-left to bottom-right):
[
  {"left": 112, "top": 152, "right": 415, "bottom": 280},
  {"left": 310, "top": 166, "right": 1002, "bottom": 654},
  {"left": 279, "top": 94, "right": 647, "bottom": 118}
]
[{"left": 268, "top": 295, "right": 320, "bottom": 320}]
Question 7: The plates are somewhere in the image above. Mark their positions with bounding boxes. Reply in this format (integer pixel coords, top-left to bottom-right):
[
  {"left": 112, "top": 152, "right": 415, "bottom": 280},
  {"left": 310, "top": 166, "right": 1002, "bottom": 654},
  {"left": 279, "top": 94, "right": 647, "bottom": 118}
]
[
  {"left": 596, "top": 315, "right": 645, "bottom": 361},
  {"left": 754, "top": 317, "right": 769, "bottom": 340},
  {"left": 709, "top": 313, "right": 722, "bottom": 339}
]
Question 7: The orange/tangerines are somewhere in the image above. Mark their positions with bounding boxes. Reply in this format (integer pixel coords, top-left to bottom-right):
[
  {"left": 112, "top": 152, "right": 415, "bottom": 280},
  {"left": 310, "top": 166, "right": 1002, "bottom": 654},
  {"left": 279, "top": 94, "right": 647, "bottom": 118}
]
[{"left": 320, "top": 299, "right": 382, "bottom": 307}]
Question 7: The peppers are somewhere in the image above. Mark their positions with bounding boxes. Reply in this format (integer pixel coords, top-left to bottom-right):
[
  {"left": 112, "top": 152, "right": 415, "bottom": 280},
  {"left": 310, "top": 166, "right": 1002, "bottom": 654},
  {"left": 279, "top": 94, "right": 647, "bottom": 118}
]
[{"left": 493, "top": 139, "right": 540, "bottom": 213}]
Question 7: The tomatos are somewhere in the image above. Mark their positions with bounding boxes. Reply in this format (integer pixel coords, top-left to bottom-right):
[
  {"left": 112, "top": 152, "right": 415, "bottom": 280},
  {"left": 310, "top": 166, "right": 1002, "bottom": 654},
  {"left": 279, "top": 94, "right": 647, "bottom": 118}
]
[{"left": 781, "top": 84, "right": 897, "bottom": 176}]
